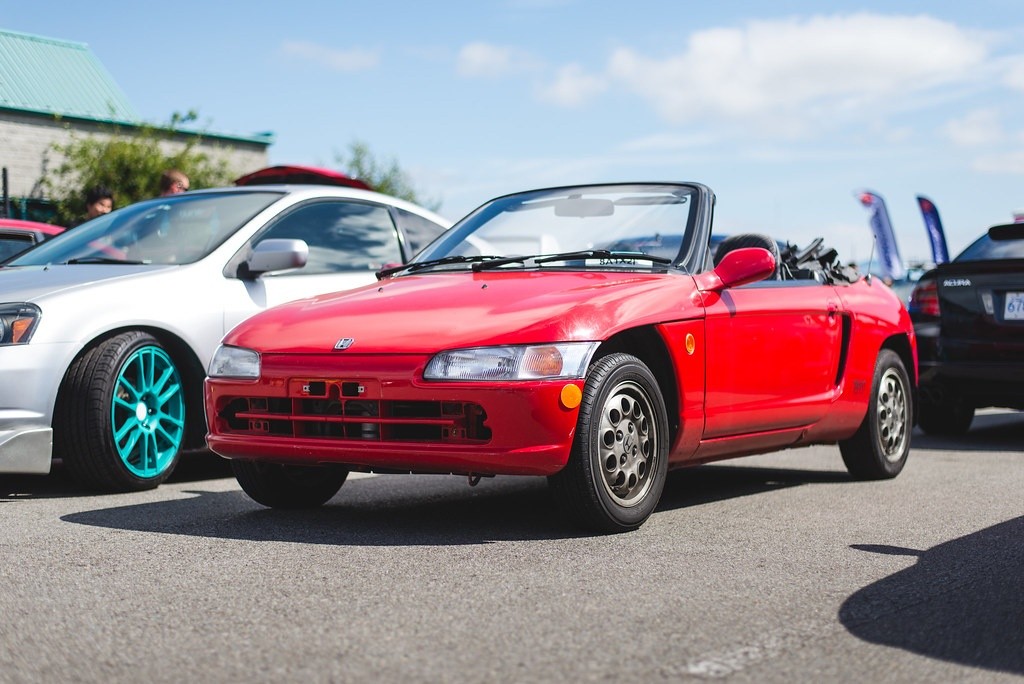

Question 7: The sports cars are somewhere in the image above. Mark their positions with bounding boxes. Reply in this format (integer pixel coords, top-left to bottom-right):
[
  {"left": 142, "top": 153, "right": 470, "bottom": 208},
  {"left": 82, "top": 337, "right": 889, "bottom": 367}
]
[{"left": 199, "top": 178, "right": 921, "bottom": 535}]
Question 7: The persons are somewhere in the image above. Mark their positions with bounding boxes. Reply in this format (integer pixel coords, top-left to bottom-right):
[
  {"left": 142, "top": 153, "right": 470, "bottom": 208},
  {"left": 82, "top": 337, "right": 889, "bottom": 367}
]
[
  {"left": 67, "top": 187, "right": 115, "bottom": 245},
  {"left": 125, "top": 169, "right": 190, "bottom": 243}
]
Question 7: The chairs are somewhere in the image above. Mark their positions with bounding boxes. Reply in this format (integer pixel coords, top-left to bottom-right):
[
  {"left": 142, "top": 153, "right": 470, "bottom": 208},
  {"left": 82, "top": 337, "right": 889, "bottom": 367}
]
[{"left": 714, "top": 234, "right": 783, "bottom": 284}]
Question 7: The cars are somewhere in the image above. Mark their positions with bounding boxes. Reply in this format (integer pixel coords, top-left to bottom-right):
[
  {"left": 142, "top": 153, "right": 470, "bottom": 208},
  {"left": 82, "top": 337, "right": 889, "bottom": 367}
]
[
  {"left": 0, "top": 180, "right": 502, "bottom": 494},
  {"left": 231, "top": 163, "right": 374, "bottom": 192},
  {"left": 0, "top": 216, "right": 127, "bottom": 267},
  {"left": 587, "top": 231, "right": 804, "bottom": 264},
  {"left": 856, "top": 257, "right": 936, "bottom": 310},
  {"left": 906, "top": 223, "right": 1024, "bottom": 440}
]
